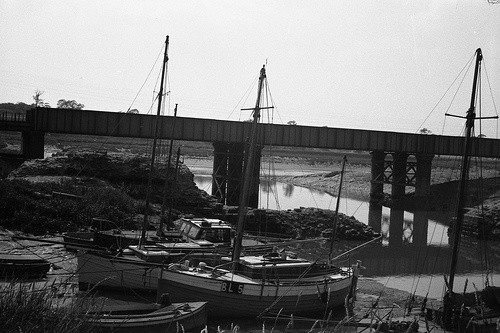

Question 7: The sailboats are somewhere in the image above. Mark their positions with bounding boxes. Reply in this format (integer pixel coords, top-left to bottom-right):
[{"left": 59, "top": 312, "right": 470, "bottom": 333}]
[
  {"left": 76, "top": 61, "right": 362, "bottom": 316},
  {"left": 62, "top": 33, "right": 272, "bottom": 254}
]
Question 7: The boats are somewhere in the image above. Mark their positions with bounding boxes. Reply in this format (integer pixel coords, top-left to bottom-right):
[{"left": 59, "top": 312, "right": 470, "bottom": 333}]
[{"left": 47, "top": 294, "right": 209, "bottom": 332}]
